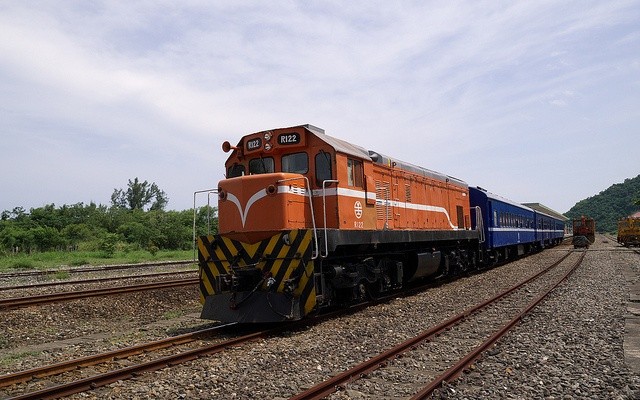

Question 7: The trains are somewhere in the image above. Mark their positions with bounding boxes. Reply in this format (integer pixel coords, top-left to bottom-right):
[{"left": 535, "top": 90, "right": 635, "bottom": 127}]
[
  {"left": 572, "top": 214, "right": 595, "bottom": 248},
  {"left": 616, "top": 216, "right": 640, "bottom": 246},
  {"left": 193, "top": 124, "right": 570, "bottom": 323}
]
